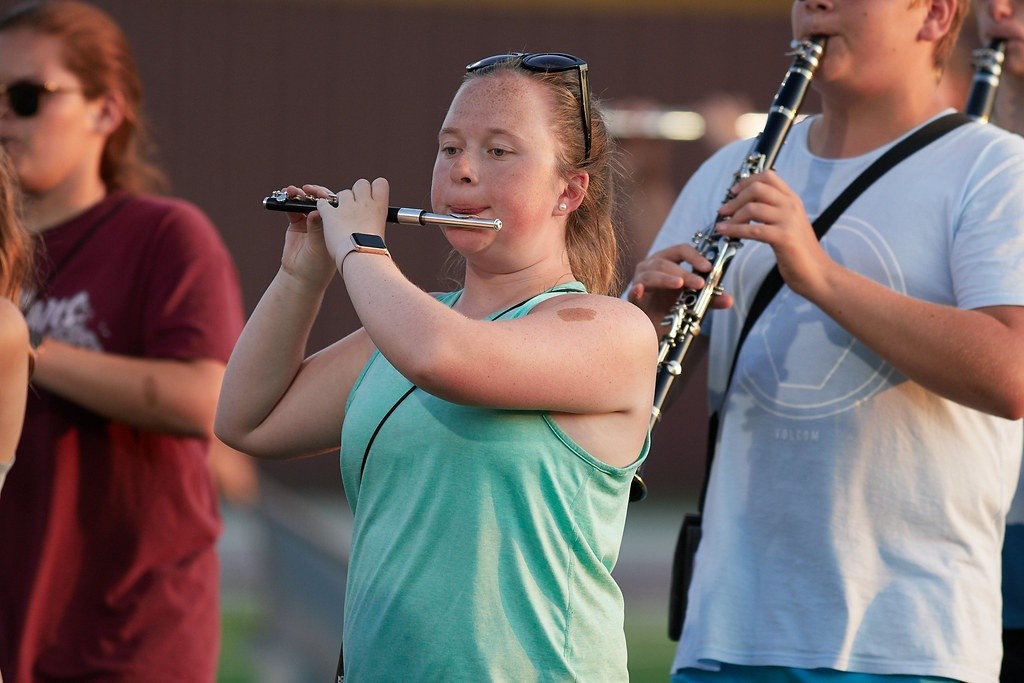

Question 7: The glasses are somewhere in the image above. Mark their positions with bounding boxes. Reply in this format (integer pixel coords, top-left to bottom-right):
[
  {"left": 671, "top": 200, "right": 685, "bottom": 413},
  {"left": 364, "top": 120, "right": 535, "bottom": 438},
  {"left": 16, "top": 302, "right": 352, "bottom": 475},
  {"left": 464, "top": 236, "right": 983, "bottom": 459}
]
[
  {"left": 462, "top": 52, "right": 596, "bottom": 165},
  {"left": 1, "top": 77, "right": 105, "bottom": 120}
]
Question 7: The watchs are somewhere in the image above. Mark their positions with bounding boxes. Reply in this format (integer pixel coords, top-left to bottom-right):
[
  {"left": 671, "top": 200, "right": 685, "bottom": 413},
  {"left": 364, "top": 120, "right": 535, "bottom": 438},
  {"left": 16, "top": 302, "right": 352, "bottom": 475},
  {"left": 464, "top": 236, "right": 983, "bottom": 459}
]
[{"left": 329, "top": 228, "right": 393, "bottom": 288}]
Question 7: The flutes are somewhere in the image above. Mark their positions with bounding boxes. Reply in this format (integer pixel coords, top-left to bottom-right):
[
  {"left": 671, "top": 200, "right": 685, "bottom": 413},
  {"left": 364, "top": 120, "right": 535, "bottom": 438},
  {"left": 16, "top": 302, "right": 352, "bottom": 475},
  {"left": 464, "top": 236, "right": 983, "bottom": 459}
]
[
  {"left": 960, "top": 36, "right": 1007, "bottom": 125},
  {"left": 262, "top": 190, "right": 502, "bottom": 231},
  {"left": 628, "top": 33, "right": 828, "bottom": 506}
]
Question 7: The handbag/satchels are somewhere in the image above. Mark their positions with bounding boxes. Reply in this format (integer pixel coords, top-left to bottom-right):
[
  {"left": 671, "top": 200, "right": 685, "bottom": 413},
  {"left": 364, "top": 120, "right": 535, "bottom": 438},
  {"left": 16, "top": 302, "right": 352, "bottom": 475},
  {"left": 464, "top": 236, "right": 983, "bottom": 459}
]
[{"left": 666, "top": 510, "right": 713, "bottom": 642}]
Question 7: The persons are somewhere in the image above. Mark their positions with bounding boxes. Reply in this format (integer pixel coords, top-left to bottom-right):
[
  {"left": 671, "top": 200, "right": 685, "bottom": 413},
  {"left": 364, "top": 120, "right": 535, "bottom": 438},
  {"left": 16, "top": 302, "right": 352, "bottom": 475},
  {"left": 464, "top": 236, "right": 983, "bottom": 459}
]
[
  {"left": 1, "top": 1, "right": 250, "bottom": 680},
  {"left": 210, "top": 46, "right": 661, "bottom": 683},
  {"left": 612, "top": 0, "right": 1023, "bottom": 680},
  {"left": 1, "top": 141, "right": 51, "bottom": 496},
  {"left": 902, "top": 24, "right": 1022, "bottom": 676},
  {"left": 685, "top": 78, "right": 759, "bottom": 154},
  {"left": 964, "top": 1, "right": 1023, "bottom": 136}
]
